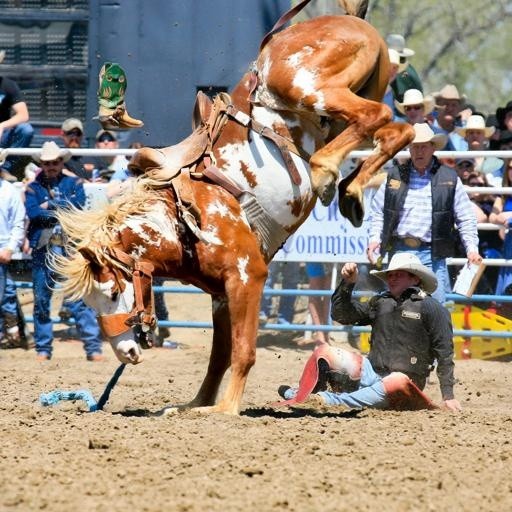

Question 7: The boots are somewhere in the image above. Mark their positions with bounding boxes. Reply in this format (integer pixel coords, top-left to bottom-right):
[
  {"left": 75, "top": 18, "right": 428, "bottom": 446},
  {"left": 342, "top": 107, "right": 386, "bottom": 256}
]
[{"left": 98, "top": 62, "right": 144, "bottom": 128}]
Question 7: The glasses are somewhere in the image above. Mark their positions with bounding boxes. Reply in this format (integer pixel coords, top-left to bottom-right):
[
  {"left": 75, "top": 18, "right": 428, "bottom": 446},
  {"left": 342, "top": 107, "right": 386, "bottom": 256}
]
[
  {"left": 44, "top": 161, "right": 59, "bottom": 166},
  {"left": 98, "top": 135, "right": 113, "bottom": 142},
  {"left": 66, "top": 132, "right": 82, "bottom": 137},
  {"left": 404, "top": 106, "right": 424, "bottom": 112}
]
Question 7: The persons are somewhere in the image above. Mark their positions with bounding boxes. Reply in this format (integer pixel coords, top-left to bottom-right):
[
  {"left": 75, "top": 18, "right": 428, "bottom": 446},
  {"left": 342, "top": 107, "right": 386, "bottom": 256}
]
[
  {"left": 258, "top": 33, "right": 512, "bottom": 348},
  {"left": 278, "top": 253, "right": 463, "bottom": 414},
  {"left": 0, "top": 50, "right": 34, "bottom": 182},
  {"left": 0, "top": 119, "right": 171, "bottom": 361}
]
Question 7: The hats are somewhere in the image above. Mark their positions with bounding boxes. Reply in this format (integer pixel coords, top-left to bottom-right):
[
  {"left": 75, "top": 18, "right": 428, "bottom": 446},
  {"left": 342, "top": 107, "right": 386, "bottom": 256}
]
[
  {"left": 62, "top": 117, "right": 83, "bottom": 132},
  {"left": 455, "top": 157, "right": 474, "bottom": 165},
  {"left": 387, "top": 32, "right": 415, "bottom": 58},
  {"left": 96, "top": 129, "right": 117, "bottom": 139},
  {"left": 433, "top": 84, "right": 512, "bottom": 139},
  {"left": 32, "top": 141, "right": 72, "bottom": 164},
  {"left": 407, "top": 122, "right": 448, "bottom": 149},
  {"left": 369, "top": 251, "right": 438, "bottom": 293},
  {"left": 394, "top": 90, "right": 435, "bottom": 117}
]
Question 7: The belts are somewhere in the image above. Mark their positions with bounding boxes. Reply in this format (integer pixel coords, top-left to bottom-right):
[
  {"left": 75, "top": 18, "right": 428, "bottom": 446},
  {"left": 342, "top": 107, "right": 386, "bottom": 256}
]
[{"left": 393, "top": 235, "right": 432, "bottom": 248}]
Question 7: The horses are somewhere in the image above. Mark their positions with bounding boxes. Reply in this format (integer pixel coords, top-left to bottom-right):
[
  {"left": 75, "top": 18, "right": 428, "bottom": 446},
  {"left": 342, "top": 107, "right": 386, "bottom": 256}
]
[{"left": 45, "top": 0, "right": 416, "bottom": 418}]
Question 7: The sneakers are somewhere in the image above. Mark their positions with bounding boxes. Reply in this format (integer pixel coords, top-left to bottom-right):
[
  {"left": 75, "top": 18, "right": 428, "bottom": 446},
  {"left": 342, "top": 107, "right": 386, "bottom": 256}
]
[
  {"left": 278, "top": 385, "right": 296, "bottom": 398},
  {"left": 88, "top": 353, "right": 103, "bottom": 362},
  {"left": 38, "top": 352, "right": 49, "bottom": 360},
  {"left": 0, "top": 332, "right": 27, "bottom": 348},
  {"left": 156, "top": 338, "right": 177, "bottom": 347}
]
